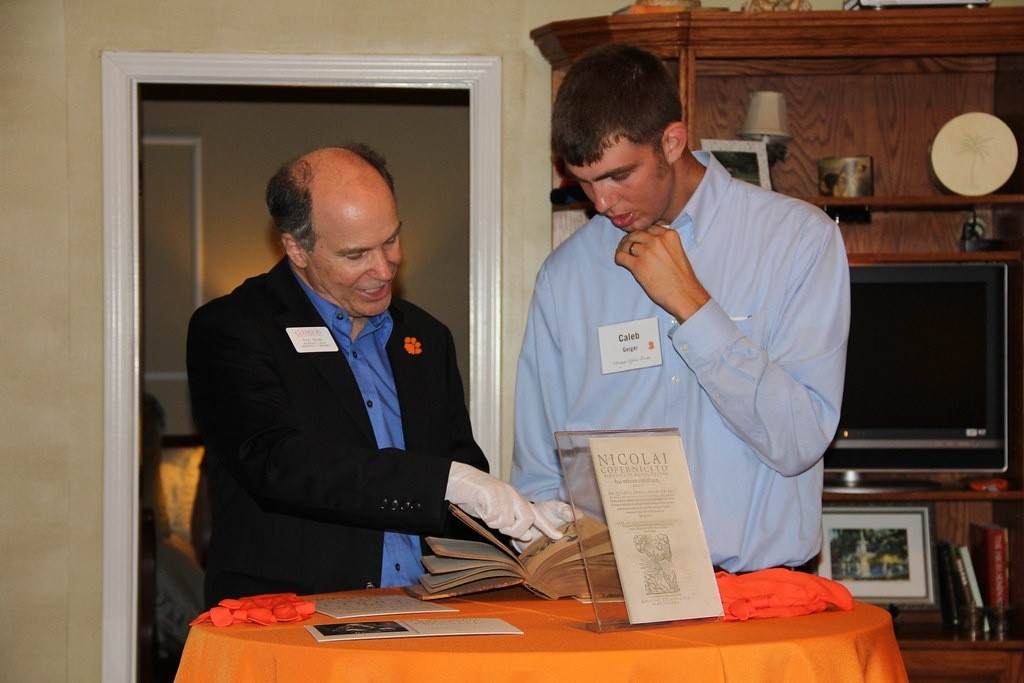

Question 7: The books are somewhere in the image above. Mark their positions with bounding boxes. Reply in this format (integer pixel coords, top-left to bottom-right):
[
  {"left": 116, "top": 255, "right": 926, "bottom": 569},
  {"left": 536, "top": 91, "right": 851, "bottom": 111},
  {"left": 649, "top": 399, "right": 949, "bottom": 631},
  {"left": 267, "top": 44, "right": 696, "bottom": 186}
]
[
  {"left": 406, "top": 499, "right": 623, "bottom": 601},
  {"left": 929, "top": 520, "right": 1012, "bottom": 640}
]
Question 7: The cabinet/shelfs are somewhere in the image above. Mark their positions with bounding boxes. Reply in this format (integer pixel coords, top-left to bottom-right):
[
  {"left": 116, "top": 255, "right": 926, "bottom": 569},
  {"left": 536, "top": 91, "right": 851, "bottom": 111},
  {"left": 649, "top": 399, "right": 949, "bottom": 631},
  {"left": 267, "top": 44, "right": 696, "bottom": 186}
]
[{"left": 529, "top": 6, "right": 1024, "bottom": 683}]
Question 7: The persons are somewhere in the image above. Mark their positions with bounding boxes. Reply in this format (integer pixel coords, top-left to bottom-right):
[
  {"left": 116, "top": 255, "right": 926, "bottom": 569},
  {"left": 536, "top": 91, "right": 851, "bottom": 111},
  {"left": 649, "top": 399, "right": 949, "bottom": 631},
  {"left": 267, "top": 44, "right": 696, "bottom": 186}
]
[
  {"left": 182, "top": 138, "right": 584, "bottom": 618},
  {"left": 500, "top": 42, "right": 853, "bottom": 577}
]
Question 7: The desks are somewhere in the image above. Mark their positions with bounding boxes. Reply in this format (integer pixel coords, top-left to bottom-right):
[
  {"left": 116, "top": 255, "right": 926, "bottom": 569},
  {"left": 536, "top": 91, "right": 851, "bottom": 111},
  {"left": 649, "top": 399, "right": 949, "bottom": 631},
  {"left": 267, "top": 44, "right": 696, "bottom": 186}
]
[{"left": 173, "top": 569, "right": 907, "bottom": 683}]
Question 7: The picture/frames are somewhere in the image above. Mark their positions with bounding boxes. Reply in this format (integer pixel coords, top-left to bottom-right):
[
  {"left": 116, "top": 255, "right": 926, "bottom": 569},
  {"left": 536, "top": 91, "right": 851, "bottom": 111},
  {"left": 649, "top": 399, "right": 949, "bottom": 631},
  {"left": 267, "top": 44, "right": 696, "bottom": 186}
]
[{"left": 812, "top": 506, "right": 934, "bottom": 605}]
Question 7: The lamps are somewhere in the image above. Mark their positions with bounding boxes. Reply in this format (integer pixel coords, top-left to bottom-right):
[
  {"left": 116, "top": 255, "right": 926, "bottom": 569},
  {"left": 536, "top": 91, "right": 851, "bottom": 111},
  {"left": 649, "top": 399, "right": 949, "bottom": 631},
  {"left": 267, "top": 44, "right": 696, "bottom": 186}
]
[{"left": 740, "top": 91, "right": 793, "bottom": 155}]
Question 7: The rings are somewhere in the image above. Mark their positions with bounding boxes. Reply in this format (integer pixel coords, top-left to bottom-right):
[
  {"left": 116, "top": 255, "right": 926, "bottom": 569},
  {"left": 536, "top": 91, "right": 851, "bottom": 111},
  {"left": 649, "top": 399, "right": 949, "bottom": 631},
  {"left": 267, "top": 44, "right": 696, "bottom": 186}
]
[{"left": 628, "top": 241, "right": 641, "bottom": 256}]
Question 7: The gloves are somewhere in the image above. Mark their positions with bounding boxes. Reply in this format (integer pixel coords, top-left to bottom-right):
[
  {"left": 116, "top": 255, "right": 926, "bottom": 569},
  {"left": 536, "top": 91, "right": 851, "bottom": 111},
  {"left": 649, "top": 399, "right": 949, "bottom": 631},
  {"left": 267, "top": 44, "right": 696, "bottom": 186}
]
[
  {"left": 187, "top": 592, "right": 317, "bottom": 627},
  {"left": 713, "top": 566, "right": 853, "bottom": 622},
  {"left": 511, "top": 498, "right": 583, "bottom": 554},
  {"left": 444, "top": 461, "right": 564, "bottom": 542}
]
